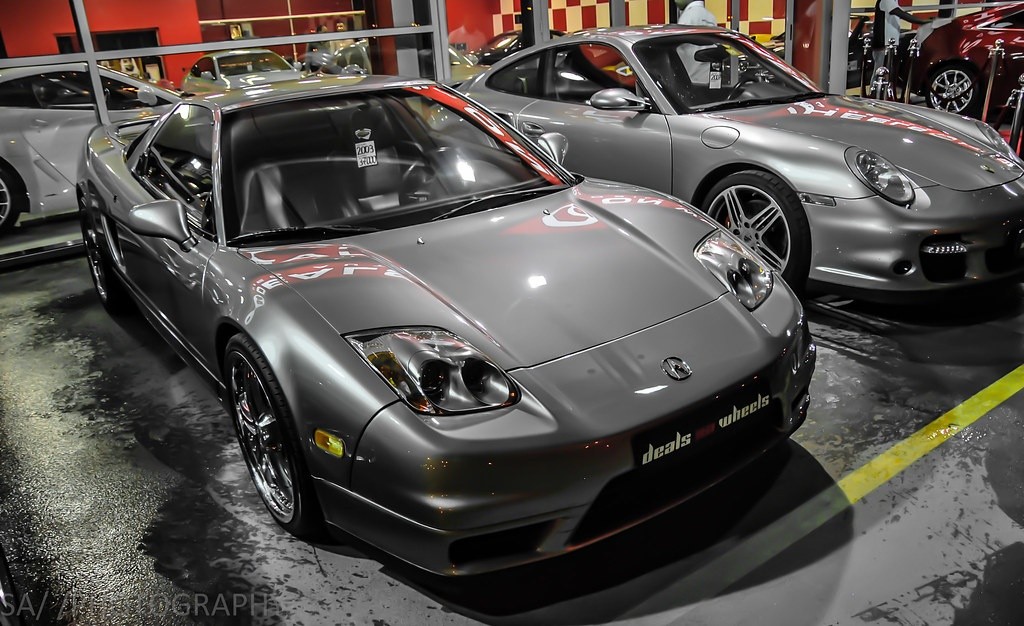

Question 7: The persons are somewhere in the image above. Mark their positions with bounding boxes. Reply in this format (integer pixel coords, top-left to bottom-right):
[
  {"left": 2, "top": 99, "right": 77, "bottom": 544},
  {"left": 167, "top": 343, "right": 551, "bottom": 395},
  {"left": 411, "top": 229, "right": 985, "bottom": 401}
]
[
  {"left": 674, "top": 0, "right": 718, "bottom": 83},
  {"left": 446, "top": 14, "right": 487, "bottom": 62},
  {"left": 304, "top": 25, "right": 330, "bottom": 76},
  {"left": 330, "top": 22, "right": 354, "bottom": 52},
  {"left": 869, "top": 0, "right": 934, "bottom": 94}
]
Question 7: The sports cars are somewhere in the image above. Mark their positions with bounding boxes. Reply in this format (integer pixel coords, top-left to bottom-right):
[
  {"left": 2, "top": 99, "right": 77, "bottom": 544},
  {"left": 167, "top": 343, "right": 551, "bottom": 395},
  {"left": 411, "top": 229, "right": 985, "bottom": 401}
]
[
  {"left": 72, "top": 72, "right": 818, "bottom": 583},
  {"left": 447, "top": 24, "right": 1024, "bottom": 299}
]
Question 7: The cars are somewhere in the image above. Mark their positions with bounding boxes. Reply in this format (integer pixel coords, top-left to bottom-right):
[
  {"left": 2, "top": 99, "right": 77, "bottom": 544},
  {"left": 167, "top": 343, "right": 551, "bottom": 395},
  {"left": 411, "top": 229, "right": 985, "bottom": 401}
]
[
  {"left": 318, "top": 36, "right": 475, "bottom": 75},
  {"left": 0, "top": 53, "right": 186, "bottom": 239},
  {"left": 181, "top": 48, "right": 306, "bottom": 95},
  {"left": 885, "top": 1, "right": 1024, "bottom": 114},
  {"left": 464, "top": 28, "right": 570, "bottom": 66}
]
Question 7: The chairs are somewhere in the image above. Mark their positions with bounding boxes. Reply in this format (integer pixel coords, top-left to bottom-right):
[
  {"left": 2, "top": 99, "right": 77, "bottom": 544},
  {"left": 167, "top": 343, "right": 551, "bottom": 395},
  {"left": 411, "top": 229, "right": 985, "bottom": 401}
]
[{"left": 332, "top": 102, "right": 427, "bottom": 220}]
